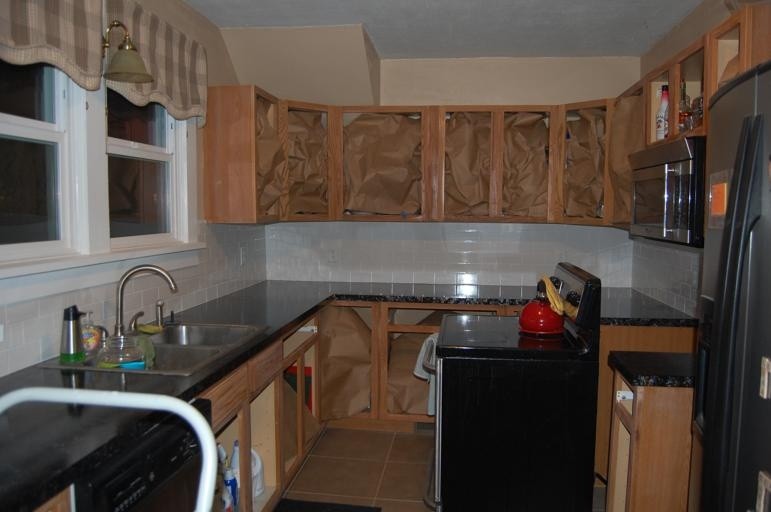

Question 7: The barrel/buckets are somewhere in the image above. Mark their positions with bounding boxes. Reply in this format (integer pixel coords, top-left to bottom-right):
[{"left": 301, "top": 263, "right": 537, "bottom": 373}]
[{"left": 228, "top": 439, "right": 264, "bottom": 498}]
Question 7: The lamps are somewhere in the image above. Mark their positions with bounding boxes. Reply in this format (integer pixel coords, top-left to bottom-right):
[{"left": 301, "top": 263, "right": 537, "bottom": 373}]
[{"left": 101, "top": 20, "right": 154, "bottom": 85}]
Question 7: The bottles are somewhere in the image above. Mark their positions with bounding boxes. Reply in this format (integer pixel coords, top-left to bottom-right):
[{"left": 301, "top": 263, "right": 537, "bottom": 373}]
[
  {"left": 96, "top": 337, "right": 145, "bottom": 370},
  {"left": 656, "top": 79, "right": 703, "bottom": 140},
  {"left": 215, "top": 441, "right": 237, "bottom": 508}
]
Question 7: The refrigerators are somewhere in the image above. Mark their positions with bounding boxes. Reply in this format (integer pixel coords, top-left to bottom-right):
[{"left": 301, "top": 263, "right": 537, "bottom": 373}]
[{"left": 687, "top": 58, "right": 770, "bottom": 512}]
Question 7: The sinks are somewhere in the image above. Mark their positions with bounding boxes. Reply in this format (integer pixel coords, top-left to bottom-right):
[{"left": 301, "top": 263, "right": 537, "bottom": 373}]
[
  {"left": 39, "top": 337, "right": 224, "bottom": 377},
  {"left": 121, "top": 318, "right": 267, "bottom": 350}
]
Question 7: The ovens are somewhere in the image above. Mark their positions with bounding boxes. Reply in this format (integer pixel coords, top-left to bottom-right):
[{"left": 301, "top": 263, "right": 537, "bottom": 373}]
[{"left": 423, "top": 358, "right": 598, "bottom": 511}]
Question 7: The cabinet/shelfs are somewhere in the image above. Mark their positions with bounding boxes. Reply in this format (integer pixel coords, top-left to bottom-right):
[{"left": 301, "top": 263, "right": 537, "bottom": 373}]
[
  {"left": 281, "top": 100, "right": 336, "bottom": 221},
  {"left": 438, "top": 108, "right": 493, "bottom": 222},
  {"left": 278, "top": 310, "right": 318, "bottom": 492},
  {"left": 649, "top": 62, "right": 673, "bottom": 150},
  {"left": 180, "top": 337, "right": 278, "bottom": 512},
  {"left": 601, "top": 353, "right": 695, "bottom": 511},
  {"left": 384, "top": 293, "right": 498, "bottom": 423},
  {"left": 198, "top": 87, "right": 278, "bottom": 222},
  {"left": 674, "top": 36, "right": 709, "bottom": 142},
  {"left": 709, "top": 14, "right": 744, "bottom": 100},
  {"left": 610, "top": 78, "right": 649, "bottom": 227},
  {"left": 492, "top": 105, "right": 558, "bottom": 223},
  {"left": 316, "top": 297, "right": 380, "bottom": 430},
  {"left": 336, "top": 108, "right": 437, "bottom": 220},
  {"left": 558, "top": 100, "right": 612, "bottom": 226}
]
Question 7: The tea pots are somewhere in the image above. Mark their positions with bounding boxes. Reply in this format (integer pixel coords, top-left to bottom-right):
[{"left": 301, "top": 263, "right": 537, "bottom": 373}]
[{"left": 518, "top": 281, "right": 564, "bottom": 334}]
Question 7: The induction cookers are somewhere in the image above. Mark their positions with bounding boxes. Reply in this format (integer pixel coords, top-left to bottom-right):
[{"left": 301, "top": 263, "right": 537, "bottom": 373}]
[{"left": 437, "top": 314, "right": 578, "bottom": 351}]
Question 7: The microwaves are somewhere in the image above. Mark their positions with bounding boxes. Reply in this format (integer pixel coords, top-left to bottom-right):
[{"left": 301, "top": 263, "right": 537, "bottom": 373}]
[{"left": 627, "top": 136, "right": 704, "bottom": 248}]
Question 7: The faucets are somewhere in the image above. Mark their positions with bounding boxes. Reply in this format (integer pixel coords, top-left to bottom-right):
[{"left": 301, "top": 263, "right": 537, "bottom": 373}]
[
  {"left": 114, "top": 263, "right": 178, "bottom": 340},
  {"left": 155, "top": 301, "right": 163, "bottom": 325}
]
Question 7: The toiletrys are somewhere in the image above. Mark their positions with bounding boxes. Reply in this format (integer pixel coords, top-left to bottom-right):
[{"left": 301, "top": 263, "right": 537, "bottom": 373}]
[{"left": 80, "top": 308, "right": 100, "bottom": 352}]
